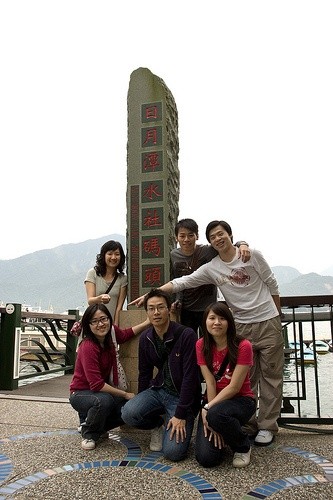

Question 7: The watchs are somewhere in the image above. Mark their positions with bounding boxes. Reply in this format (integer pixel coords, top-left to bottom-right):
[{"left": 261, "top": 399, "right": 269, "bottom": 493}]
[{"left": 204, "top": 404, "right": 210, "bottom": 409}]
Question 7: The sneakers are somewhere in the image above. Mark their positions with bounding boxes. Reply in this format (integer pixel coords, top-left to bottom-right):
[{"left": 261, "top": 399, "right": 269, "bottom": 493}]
[
  {"left": 78, "top": 422, "right": 87, "bottom": 433},
  {"left": 81, "top": 436, "right": 95, "bottom": 449},
  {"left": 232, "top": 445, "right": 251, "bottom": 468},
  {"left": 150, "top": 424, "right": 166, "bottom": 451},
  {"left": 254, "top": 429, "right": 275, "bottom": 447}
]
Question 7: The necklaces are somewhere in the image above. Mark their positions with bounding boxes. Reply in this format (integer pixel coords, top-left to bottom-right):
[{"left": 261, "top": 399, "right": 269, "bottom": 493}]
[{"left": 184, "top": 252, "right": 194, "bottom": 270}]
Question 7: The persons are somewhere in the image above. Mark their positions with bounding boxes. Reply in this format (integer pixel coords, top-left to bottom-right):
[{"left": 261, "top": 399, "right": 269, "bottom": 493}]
[
  {"left": 127, "top": 221, "right": 284, "bottom": 446},
  {"left": 170, "top": 219, "right": 252, "bottom": 339},
  {"left": 122, "top": 289, "right": 203, "bottom": 462},
  {"left": 195, "top": 302, "right": 257, "bottom": 468},
  {"left": 70, "top": 304, "right": 152, "bottom": 450},
  {"left": 76, "top": 240, "right": 128, "bottom": 353}
]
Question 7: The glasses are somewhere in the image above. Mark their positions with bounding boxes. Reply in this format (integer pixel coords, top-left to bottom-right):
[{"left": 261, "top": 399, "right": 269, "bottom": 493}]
[{"left": 89, "top": 316, "right": 109, "bottom": 326}]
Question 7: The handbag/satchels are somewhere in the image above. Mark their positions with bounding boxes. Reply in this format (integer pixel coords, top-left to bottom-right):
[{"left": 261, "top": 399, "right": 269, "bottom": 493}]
[
  {"left": 70, "top": 317, "right": 82, "bottom": 337},
  {"left": 109, "top": 325, "right": 130, "bottom": 392}
]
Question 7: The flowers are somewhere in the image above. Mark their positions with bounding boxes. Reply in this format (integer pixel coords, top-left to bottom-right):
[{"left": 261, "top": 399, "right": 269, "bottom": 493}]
[{"left": 70, "top": 316, "right": 83, "bottom": 337}]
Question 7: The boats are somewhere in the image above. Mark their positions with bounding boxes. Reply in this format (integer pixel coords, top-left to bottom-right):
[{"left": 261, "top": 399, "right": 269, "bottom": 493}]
[
  {"left": 328, "top": 340, "right": 333, "bottom": 353},
  {"left": 310, "top": 340, "right": 329, "bottom": 354},
  {"left": 283, "top": 341, "right": 319, "bottom": 365}
]
